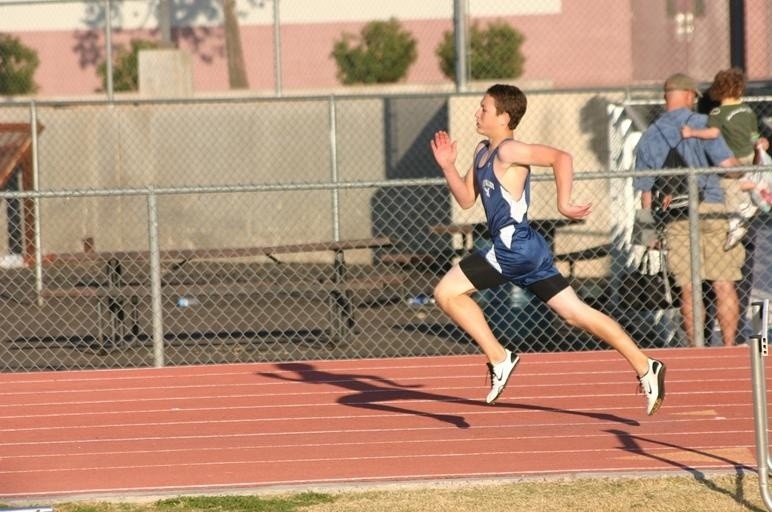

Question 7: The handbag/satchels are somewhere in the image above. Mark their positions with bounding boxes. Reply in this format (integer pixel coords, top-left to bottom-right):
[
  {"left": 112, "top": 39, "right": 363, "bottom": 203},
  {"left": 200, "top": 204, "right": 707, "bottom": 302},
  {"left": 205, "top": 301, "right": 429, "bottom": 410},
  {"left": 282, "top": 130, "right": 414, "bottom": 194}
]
[
  {"left": 624, "top": 248, "right": 680, "bottom": 346},
  {"left": 619, "top": 244, "right": 683, "bottom": 311}
]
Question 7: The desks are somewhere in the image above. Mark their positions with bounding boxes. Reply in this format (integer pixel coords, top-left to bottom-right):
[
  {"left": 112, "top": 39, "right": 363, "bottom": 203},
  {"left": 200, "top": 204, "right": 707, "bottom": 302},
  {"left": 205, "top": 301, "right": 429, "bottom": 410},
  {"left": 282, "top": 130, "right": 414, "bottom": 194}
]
[
  {"left": 429, "top": 217, "right": 587, "bottom": 308},
  {"left": 42, "top": 235, "right": 392, "bottom": 343}
]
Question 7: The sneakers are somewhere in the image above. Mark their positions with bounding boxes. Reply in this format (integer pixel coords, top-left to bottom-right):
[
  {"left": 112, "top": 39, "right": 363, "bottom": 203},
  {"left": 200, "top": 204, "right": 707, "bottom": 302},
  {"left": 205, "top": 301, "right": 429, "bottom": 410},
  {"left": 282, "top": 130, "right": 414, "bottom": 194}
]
[
  {"left": 636, "top": 357, "right": 667, "bottom": 416},
  {"left": 722, "top": 224, "right": 748, "bottom": 252},
  {"left": 740, "top": 203, "right": 759, "bottom": 226},
  {"left": 485, "top": 348, "right": 520, "bottom": 405}
]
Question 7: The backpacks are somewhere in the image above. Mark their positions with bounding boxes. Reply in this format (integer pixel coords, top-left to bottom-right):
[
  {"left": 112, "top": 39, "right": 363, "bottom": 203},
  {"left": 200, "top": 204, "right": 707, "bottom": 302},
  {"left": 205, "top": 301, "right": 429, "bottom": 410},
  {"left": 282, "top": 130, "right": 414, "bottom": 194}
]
[{"left": 650, "top": 114, "right": 707, "bottom": 221}]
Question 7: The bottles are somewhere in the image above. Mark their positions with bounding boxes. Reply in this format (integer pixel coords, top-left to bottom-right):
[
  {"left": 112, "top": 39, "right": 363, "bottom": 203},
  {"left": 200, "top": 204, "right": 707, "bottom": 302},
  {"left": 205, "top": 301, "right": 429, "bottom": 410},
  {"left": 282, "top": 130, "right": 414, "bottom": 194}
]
[
  {"left": 175, "top": 297, "right": 202, "bottom": 308},
  {"left": 406, "top": 297, "right": 436, "bottom": 306}
]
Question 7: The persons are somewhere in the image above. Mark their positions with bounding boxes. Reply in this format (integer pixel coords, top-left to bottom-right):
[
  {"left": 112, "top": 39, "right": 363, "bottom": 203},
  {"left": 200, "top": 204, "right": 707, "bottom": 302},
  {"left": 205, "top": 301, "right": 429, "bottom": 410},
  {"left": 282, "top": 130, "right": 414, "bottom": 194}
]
[
  {"left": 694, "top": 86, "right": 771, "bottom": 167},
  {"left": 428, "top": 83, "right": 667, "bottom": 416},
  {"left": 633, "top": 69, "right": 746, "bottom": 348},
  {"left": 680, "top": 64, "right": 761, "bottom": 251}
]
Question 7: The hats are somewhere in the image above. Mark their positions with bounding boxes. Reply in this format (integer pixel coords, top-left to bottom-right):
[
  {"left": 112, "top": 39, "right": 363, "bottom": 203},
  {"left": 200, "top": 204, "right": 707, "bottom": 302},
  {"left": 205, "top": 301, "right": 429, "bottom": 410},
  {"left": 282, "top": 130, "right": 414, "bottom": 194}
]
[{"left": 663, "top": 73, "right": 703, "bottom": 98}]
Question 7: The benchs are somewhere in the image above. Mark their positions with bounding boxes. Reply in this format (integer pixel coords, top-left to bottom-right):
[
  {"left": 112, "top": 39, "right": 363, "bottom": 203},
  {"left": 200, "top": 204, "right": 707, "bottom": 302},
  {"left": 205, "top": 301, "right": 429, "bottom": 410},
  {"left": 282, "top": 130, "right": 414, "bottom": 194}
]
[
  {"left": 41, "top": 270, "right": 404, "bottom": 355},
  {"left": 381, "top": 253, "right": 474, "bottom": 305}
]
